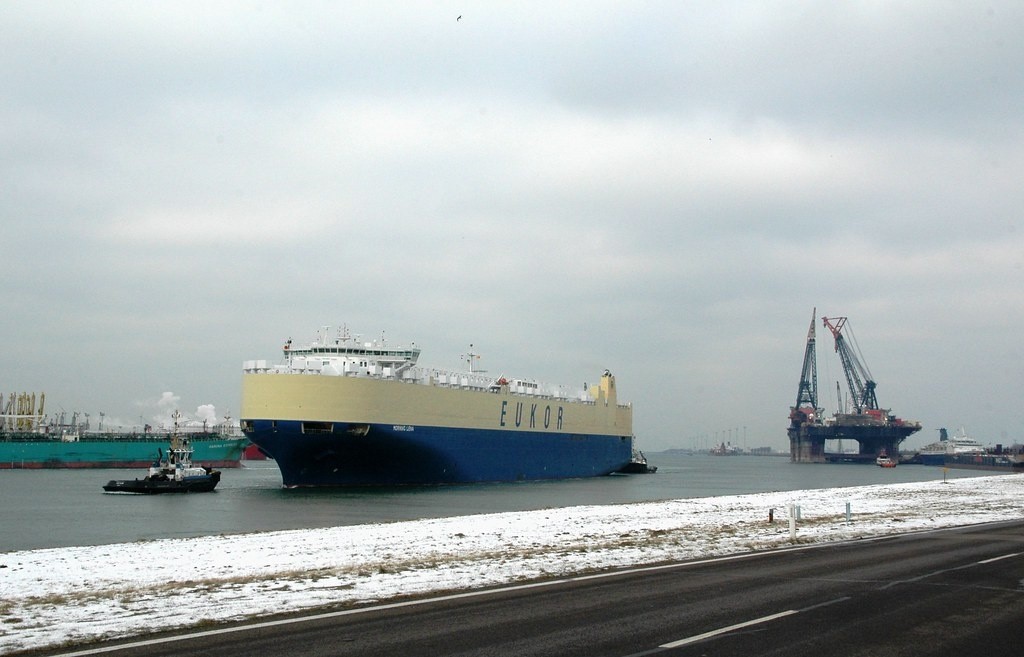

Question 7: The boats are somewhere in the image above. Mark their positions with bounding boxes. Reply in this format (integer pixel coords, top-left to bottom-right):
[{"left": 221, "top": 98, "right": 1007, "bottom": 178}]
[
  {"left": 709, "top": 442, "right": 734, "bottom": 456},
  {"left": 0, "top": 391, "right": 254, "bottom": 469},
  {"left": 920, "top": 426, "right": 986, "bottom": 466},
  {"left": 984, "top": 443, "right": 1024, "bottom": 471},
  {"left": 876, "top": 447, "right": 896, "bottom": 468},
  {"left": 242, "top": 443, "right": 267, "bottom": 461},
  {"left": 615, "top": 434, "right": 658, "bottom": 474},
  {"left": 103, "top": 409, "right": 222, "bottom": 493}
]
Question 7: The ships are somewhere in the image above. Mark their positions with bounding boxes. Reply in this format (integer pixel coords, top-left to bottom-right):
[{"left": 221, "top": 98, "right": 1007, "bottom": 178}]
[{"left": 239, "top": 323, "right": 633, "bottom": 488}]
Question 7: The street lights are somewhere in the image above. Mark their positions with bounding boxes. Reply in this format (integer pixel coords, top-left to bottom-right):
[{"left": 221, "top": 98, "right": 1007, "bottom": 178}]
[
  {"left": 715, "top": 432, "right": 717, "bottom": 442},
  {"left": 728, "top": 430, "right": 732, "bottom": 442},
  {"left": 743, "top": 426, "right": 746, "bottom": 450},
  {"left": 735, "top": 428, "right": 738, "bottom": 445},
  {"left": 723, "top": 430, "right": 725, "bottom": 442}
]
